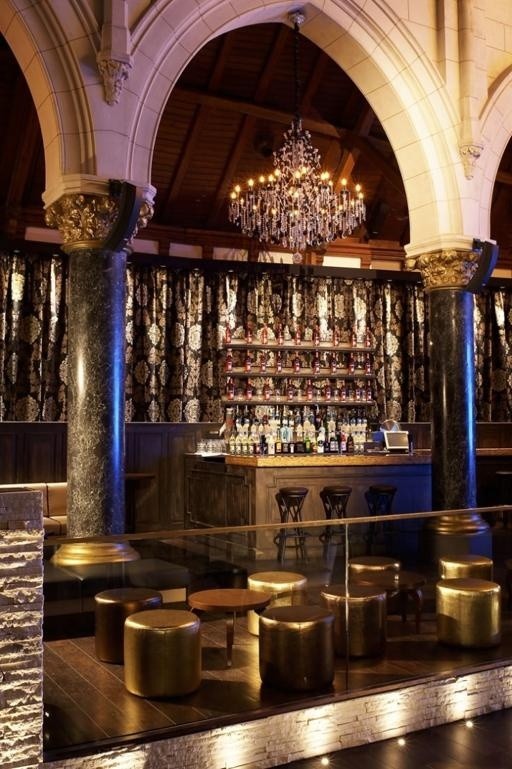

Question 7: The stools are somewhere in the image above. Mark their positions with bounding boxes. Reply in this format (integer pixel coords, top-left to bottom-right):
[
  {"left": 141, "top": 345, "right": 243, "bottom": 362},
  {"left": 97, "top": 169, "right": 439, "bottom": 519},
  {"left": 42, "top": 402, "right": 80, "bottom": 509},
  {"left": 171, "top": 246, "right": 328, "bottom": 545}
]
[
  {"left": 322, "top": 582, "right": 387, "bottom": 655},
  {"left": 118, "top": 471, "right": 159, "bottom": 558},
  {"left": 436, "top": 553, "right": 494, "bottom": 581},
  {"left": 94, "top": 587, "right": 162, "bottom": 664},
  {"left": 123, "top": 610, "right": 202, "bottom": 699},
  {"left": 258, "top": 604, "right": 335, "bottom": 696},
  {"left": 278, "top": 484, "right": 397, "bottom": 562},
  {"left": 247, "top": 571, "right": 308, "bottom": 635},
  {"left": 349, "top": 555, "right": 400, "bottom": 615},
  {"left": 435, "top": 579, "right": 500, "bottom": 652}
]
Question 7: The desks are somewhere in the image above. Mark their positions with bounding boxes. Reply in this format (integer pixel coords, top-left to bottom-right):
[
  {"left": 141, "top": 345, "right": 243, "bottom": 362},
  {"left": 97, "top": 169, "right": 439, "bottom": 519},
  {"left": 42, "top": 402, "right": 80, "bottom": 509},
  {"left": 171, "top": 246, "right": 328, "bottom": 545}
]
[
  {"left": 188, "top": 588, "right": 272, "bottom": 668},
  {"left": 357, "top": 570, "right": 427, "bottom": 634}
]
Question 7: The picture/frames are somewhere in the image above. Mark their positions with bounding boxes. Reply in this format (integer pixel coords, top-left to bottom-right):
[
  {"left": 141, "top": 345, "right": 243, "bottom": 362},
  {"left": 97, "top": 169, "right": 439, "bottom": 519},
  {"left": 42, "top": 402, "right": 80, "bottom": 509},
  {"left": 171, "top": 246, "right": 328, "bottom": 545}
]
[{"left": 383, "top": 431, "right": 411, "bottom": 450}]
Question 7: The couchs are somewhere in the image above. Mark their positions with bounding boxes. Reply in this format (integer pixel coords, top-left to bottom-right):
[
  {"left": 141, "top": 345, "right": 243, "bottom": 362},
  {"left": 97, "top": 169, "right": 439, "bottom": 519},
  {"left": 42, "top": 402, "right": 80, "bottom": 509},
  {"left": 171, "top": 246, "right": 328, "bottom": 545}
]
[{"left": 0, "top": 479, "right": 71, "bottom": 570}]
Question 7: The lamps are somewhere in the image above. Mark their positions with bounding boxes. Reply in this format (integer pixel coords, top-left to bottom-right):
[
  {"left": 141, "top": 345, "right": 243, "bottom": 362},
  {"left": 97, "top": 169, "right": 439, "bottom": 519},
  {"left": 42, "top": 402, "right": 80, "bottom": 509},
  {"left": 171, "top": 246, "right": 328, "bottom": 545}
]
[{"left": 227, "top": 10, "right": 368, "bottom": 266}]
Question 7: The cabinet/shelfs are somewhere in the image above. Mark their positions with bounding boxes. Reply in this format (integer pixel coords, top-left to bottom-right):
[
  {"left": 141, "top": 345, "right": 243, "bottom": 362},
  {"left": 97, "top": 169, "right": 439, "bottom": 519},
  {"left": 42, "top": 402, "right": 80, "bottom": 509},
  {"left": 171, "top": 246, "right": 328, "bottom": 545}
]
[{"left": 222, "top": 343, "right": 376, "bottom": 407}]
